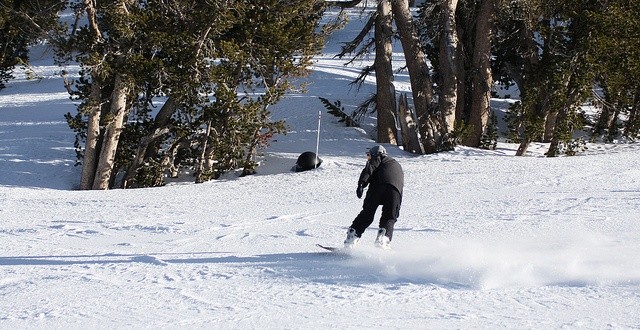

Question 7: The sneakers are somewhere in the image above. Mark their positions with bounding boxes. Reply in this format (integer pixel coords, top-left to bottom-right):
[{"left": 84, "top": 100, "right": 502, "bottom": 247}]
[
  {"left": 344, "top": 228, "right": 359, "bottom": 244},
  {"left": 375, "top": 228, "right": 390, "bottom": 247}
]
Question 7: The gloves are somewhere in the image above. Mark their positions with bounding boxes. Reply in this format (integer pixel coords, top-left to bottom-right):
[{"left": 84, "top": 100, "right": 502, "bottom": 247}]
[{"left": 357, "top": 184, "right": 363, "bottom": 198}]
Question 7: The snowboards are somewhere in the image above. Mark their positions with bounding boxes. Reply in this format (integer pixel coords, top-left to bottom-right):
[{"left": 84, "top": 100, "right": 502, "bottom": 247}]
[{"left": 317, "top": 244, "right": 338, "bottom": 252}]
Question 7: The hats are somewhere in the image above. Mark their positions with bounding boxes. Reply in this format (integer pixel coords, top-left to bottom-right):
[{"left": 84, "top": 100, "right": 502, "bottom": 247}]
[{"left": 369, "top": 145, "right": 386, "bottom": 154}]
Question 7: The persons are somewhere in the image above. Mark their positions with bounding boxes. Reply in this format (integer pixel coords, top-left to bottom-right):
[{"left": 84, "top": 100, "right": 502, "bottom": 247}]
[{"left": 343, "top": 145, "right": 404, "bottom": 249}]
